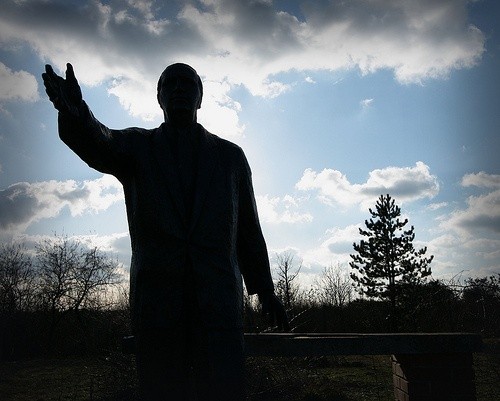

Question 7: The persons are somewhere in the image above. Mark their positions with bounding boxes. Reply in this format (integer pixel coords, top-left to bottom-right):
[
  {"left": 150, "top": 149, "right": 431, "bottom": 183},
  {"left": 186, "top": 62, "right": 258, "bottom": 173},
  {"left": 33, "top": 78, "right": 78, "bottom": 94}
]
[{"left": 41, "top": 63, "right": 290, "bottom": 401}]
